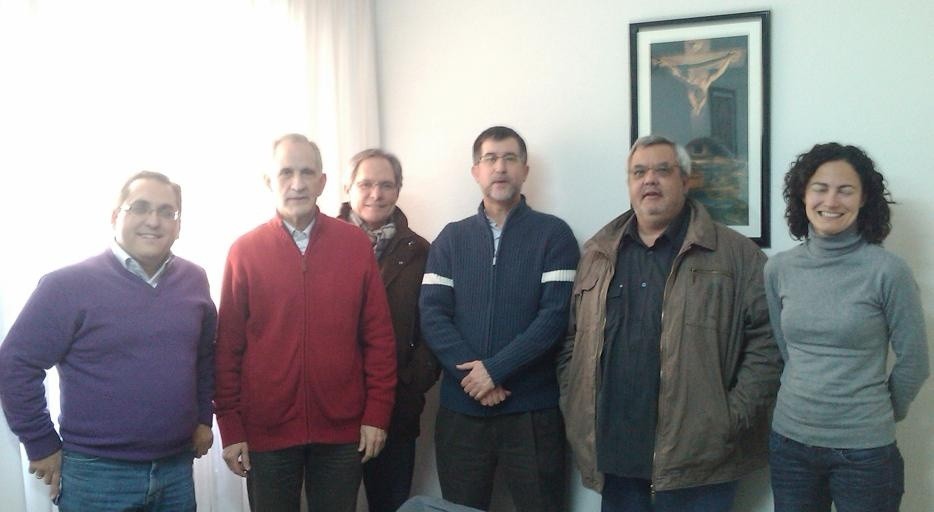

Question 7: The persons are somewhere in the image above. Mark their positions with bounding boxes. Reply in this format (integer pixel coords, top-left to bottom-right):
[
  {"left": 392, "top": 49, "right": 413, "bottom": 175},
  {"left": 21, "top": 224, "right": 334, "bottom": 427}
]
[
  {"left": 415, "top": 125, "right": 582, "bottom": 512},
  {"left": 761, "top": 140, "right": 930, "bottom": 510},
  {"left": 556, "top": 136, "right": 784, "bottom": 511},
  {"left": 335, "top": 147, "right": 442, "bottom": 512},
  {"left": 0, "top": 169, "right": 218, "bottom": 511},
  {"left": 214, "top": 131, "right": 398, "bottom": 511}
]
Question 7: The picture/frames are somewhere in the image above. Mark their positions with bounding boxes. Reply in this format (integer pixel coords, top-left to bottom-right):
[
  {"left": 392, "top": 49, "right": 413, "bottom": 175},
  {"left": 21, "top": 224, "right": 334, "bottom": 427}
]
[{"left": 627, "top": 9, "right": 772, "bottom": 248}]
